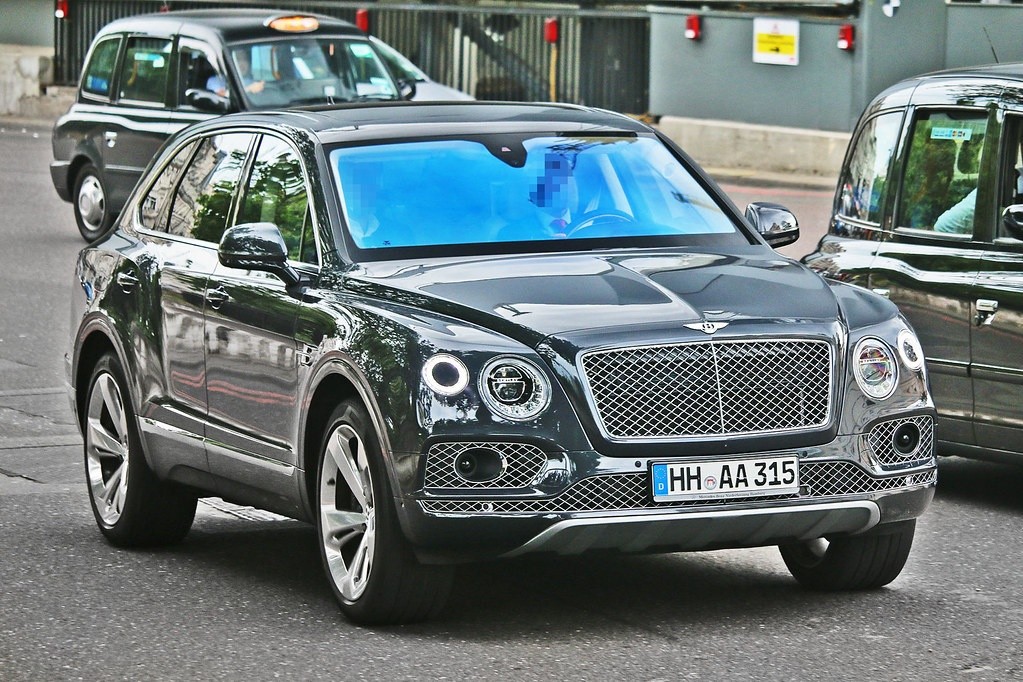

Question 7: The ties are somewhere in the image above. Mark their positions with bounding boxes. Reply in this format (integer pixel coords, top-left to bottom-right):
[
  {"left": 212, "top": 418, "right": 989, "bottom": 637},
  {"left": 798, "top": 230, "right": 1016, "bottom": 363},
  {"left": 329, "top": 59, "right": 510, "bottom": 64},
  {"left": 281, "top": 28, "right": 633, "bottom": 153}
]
[{"left": 551, "top": 219, "right": 567, "bottom": 233}]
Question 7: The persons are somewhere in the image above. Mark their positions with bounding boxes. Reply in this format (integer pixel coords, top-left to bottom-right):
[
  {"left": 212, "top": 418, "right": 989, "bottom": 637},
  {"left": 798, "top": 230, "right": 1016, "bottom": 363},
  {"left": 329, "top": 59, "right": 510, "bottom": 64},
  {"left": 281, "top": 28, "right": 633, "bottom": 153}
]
[
  {"left": 933, "top": 188, "right": 976, "bottom": 236},
  {"left": 498, "top": 164, "right": 633, "bottom": 241},
  {"left": 205, "top": 50, "right": 264, "bottom": 99}
]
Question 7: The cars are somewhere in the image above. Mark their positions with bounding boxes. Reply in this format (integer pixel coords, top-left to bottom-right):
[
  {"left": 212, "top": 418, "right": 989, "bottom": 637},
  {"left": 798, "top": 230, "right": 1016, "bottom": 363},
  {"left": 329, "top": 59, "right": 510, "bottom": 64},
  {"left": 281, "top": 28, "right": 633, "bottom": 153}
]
[
  {"left": 61, "top": 100, "right": 939, "bottom": 629},
  {"left": 49, "top": 6, "right": 416, "bottom": 246},
  {"left": 251, "top": 36, "right": 477, "bottom": 103},
  {"left": 800, "top": 62, "right": 1023, "bottom": 472}
]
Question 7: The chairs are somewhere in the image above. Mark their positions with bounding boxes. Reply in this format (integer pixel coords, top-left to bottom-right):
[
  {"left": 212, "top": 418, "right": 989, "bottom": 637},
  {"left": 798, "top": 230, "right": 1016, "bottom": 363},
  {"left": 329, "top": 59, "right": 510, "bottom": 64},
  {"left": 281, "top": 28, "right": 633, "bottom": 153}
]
[
  {"left": 942, "top": 132, "right": 985, "bottom": 215},
  {"left": 360, "top": 172, "right": 462, "bottom": 248}
]
